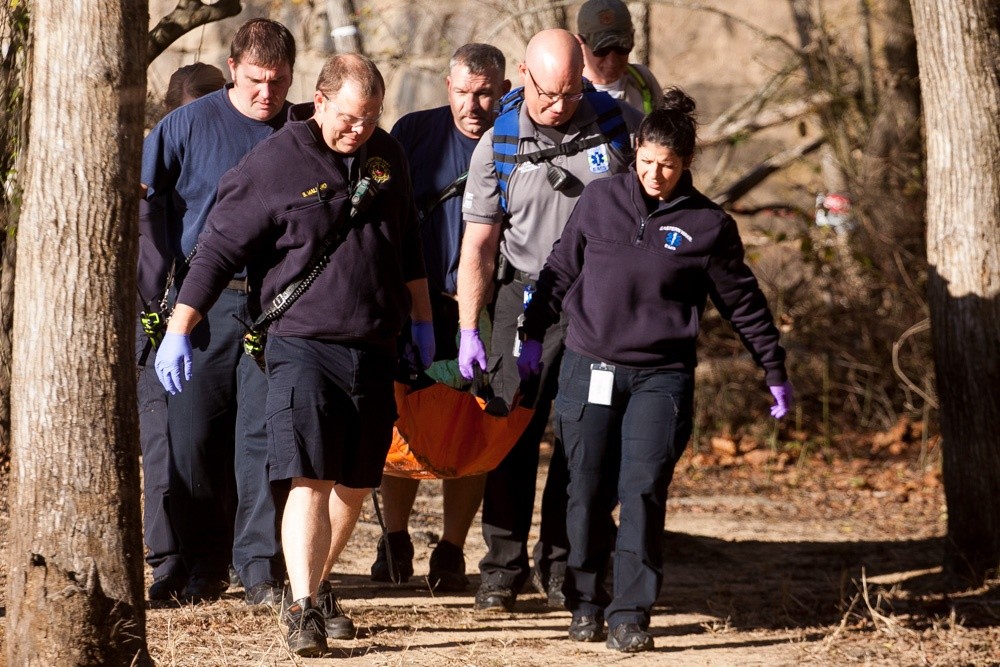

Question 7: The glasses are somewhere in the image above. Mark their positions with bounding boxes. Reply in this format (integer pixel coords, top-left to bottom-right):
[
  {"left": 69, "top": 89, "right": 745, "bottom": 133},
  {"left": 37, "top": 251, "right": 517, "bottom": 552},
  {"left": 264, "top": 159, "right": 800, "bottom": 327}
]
[
  {"left": 579, "top": 36, "right": 631, "bottom": 58},
  {"left": 316, "top": 87, "right": 384, "bottom": 126},
  {"left": 524, "top": 60, "right": 584, "bottom": 103}
]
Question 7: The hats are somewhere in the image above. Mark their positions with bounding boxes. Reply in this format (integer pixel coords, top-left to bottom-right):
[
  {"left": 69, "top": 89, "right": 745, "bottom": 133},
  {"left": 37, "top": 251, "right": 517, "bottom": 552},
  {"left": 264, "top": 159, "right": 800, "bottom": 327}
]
[{"left": 577, "top": 0, "right": 634, "bottom": 52}]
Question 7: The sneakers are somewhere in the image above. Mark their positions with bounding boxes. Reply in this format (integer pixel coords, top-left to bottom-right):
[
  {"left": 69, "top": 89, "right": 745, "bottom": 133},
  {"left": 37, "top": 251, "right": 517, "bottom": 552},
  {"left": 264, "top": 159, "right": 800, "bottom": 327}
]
[
  {"left": 148, "top": 573, "right": 231, "bottom": 604},
  {"left": 229, "top": 565, "right": 242, "bottom": 587},
  {"left": 530, "top": 564, "right": 566, "bottom": 609},
  {"left": 606, "top": 622, "right": 654, "bottom": 654},
  {"left": 569, "top": 612, "right": 604, "bottom": 643},
  {"left": 427, "top": 537, "right": 468, "bottom": 592},
  {"left": 372, "top": 532, "right": 414, "bottom": 582},
  {"left": 244, "top": 579, "right": 288, "bottom": 605},
  {"left": 286, "top": 596, "right": 327, "bottom": 657},
  {"left": 472, "top": 581, "right": 517, "bottom": 620},
  {"left": 315, "top": 581, "right": 355, "bottom": 640}
]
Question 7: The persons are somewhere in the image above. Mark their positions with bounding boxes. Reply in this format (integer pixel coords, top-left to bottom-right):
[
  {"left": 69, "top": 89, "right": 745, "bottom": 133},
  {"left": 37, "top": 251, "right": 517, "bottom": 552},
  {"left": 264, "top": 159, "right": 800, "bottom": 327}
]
[
  {"left": 518, "top": 88, "right": 788, "bottom": 651},
  {"left": 154, "top": 51, "right": 436, "bottom": 656},
  {"left": 132, "top": 0, "right": 673, "bottom": 604},
  {"left": 457, "top": 29, "right": 646, "bottom": 614}
]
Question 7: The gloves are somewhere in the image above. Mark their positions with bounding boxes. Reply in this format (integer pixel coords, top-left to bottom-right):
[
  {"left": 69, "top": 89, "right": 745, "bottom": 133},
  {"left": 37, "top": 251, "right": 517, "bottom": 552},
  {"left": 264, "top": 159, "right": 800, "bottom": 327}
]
[
  {"left": 154, "top": 333, "right": 193, "bottom": 396},
  {"left": 769, "top": 379, "right": 793, "bottom": 420},
  {"left": 406, "top": 322, "right": 436, "bottom": 369},
  {"left": 516, "top": 339, "right": 543, "bottom": 382},
  {"left": 458, "top": 330, "right": 487, "bottom": 380}
]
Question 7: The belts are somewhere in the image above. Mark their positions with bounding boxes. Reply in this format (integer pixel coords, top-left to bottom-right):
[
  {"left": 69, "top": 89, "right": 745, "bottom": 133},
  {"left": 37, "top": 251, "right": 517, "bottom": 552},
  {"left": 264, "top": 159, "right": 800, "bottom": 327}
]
[
  {"left": 224, "top": 276, "right": 252, "bottom": 293},
  {"left": 510, "top": 267, "right": 536, "bottom": 289}
]
